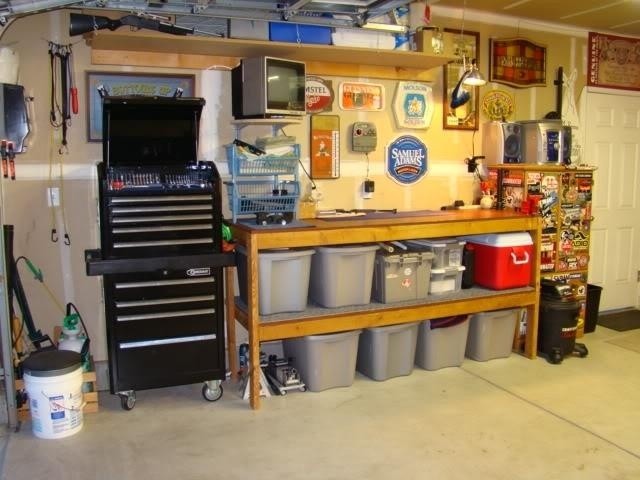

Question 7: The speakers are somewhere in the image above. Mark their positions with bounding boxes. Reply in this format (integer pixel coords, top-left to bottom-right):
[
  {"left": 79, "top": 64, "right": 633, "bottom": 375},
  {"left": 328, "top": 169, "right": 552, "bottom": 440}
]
[
  {"left": 562, "top": 126, "right": 572, "bottom": 162},
  {"left": 483, "top": 119, "right": 521, "bottom": 165}
]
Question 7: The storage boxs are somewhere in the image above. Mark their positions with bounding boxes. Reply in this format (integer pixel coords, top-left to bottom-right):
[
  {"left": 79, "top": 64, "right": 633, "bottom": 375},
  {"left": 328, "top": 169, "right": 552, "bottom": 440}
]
[
  {"left": 371, "top": 237, "right": 466, "bottom": 304},
  {"left": 355, "top": 321, "right": 421, "bottom": 382},
  {"left": 235, "top": 241, "right": 316, "bottom": 316},
  {"left": 308, "top": 244, "right": 381, "bottom": 308},
  {"left": 282, "top": 329, "right": 364, "bottom": 393},
  {"left": 414, "top": 313, "right": 474, "bottom": 371},
  {"left": 465, "top": 307, "right": 522, "bottom": 362},
  {"left": 457, "top": 231, "right": 536, "bottom": 290}
]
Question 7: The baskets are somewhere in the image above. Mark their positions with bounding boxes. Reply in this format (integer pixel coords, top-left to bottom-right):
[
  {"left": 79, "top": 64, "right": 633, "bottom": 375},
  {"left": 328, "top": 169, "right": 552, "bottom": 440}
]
[
  {"left": 223, "top": 142, "right": 299, "bottom": 177},
  {"left": 225, "top": 180, "right": 300, "bottom": 215}
]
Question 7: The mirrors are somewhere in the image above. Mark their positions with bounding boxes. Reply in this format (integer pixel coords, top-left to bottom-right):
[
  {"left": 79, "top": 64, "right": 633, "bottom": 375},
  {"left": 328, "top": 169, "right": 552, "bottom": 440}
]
[{"left": 442, "top": 28, "right": 481, "bottom": 131}]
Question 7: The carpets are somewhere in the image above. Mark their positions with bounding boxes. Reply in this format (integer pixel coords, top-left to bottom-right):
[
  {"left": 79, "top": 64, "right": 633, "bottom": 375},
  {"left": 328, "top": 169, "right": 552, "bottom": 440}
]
[{"left": 596, "top": 309, "right": 640, "bottom": 332}]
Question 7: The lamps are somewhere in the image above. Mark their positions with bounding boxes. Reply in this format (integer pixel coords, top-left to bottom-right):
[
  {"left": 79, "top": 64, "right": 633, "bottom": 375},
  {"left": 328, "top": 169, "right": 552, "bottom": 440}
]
[{"left": 450, "top": 58, "right": 488, "bottom": 109}]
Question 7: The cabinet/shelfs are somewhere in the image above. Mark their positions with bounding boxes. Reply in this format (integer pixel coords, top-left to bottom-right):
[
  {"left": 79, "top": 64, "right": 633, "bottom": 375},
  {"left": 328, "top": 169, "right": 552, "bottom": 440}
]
[
  {"left": 222, "top": 143, "right": 302, "bottom": 224},
  {"left": 488, "top": 164, "right": 600, "bottom": 338},
  {"left": 222, "top": 208, "right": 544, "bottom": 410}
]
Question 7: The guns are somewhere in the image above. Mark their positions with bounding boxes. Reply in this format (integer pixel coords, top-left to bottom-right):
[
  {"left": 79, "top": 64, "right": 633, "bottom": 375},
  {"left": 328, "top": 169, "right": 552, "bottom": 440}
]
[{"left": 70, "top": 13, "right": 194, "bottom": 36}]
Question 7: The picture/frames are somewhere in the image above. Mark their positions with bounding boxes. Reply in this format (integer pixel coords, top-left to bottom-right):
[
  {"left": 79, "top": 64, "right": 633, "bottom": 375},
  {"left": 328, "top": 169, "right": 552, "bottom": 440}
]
[
  {"left": 488, "top": 36, "right": 548, "bottom": 89},
  {"left": 384, "top": 80, "right": 435, "bottom": 187},
  {"left": 305, "top": 75, "right": 386, "bottom": 115}
]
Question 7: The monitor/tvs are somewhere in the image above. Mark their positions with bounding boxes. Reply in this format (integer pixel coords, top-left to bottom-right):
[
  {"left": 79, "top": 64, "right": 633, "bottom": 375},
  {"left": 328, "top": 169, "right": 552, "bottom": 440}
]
[
  {"left": 104, "top": 103, "right": 198, "bottom": 169},
  {"left": 230, "top": 54, "right": 307, "bottom": 117}
]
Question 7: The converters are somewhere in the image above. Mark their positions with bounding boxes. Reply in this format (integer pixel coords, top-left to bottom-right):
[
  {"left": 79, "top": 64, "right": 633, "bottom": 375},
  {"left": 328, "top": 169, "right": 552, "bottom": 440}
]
[{"left": 365, "top": 181, "right": 375, "bottom": 192}]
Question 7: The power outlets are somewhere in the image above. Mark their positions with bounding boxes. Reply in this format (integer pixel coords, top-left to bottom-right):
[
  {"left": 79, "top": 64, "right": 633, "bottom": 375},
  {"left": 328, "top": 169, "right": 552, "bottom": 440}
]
[{"left": 362, "top": 180, "right": 375, "bottom": 199}]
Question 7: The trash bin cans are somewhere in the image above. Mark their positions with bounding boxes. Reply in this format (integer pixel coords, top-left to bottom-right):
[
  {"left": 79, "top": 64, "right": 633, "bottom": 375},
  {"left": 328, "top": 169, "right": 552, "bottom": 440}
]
[
  {"left": 542, "top": 301, "right": 591, "bottom": 362},
  {"left": 585, "top": 282, "right": 604, "bottom": 332}
]
